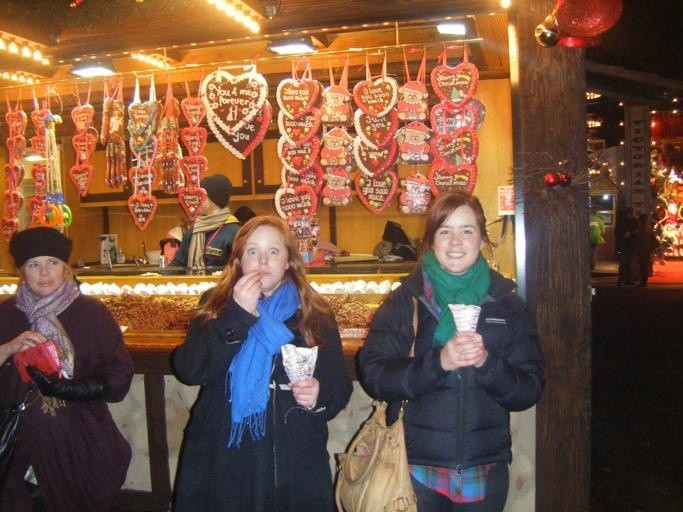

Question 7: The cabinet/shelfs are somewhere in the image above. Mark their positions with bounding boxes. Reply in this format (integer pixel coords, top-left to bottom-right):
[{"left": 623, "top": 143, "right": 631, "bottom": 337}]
[{"left": 78, "top": 128, "right": 285, "bottom": 201}]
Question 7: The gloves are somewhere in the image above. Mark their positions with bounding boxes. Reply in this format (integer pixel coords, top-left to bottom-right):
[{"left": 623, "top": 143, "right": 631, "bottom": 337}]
[{"left": 27, "top": 365, "right": 109, "bottom": 402}]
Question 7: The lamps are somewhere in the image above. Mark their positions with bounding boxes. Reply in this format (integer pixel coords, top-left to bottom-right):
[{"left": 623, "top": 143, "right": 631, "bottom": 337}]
[
  {"left": 65, "top": 60, "right": 118, "bottom": 80},
  {"left": 266, "top": 37, "right": 317, "bottom": 56}
]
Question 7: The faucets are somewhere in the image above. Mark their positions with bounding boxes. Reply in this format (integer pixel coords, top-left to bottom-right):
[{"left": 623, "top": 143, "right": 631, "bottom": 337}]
[{"left": 141, "top": 241, "right": 147, "bottom": 266}]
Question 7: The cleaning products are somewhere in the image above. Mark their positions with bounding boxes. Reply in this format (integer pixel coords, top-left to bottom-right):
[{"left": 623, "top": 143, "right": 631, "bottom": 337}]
[
  {"left": 116, "top": 246, "right": 126, "bottom": 263},
  {"left": 108, "top": 235, "right": 118, "bottom": 264}
]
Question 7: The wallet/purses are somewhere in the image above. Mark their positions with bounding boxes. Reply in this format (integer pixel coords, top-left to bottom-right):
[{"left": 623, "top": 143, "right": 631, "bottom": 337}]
[{"left": 13, "top": 340, "right": 62, "bottom": 385}]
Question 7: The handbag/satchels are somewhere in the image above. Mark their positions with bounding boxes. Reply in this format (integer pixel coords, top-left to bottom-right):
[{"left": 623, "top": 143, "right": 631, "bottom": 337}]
[
  {"left": 336, "top": 400, "right": 419, "bottom": 511},
  {"left": 0, "top": 403, "right": 31, "bottom": 477}
]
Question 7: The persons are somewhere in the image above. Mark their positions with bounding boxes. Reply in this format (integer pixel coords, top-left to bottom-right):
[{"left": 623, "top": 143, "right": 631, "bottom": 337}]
[
  {"left": 354, "top": 190, "right": 546, "bottom": 511},
  {"left": 633, "top": 213, "right": 654, "bottom": 288},
  {"left": 615, "top": 206, "right": 638, "bottom": 287},
  {"left": 159, "top": 216, "right": 190, "bottom": 268},
  {"left": 589, "top": 208, "right": 606, "bottom": 275},
  {"left": 140, "top": 172, "right": 242, "bottom": 277},
  {"left": 0, "top": 224, "right": 138, "bottom": 511},
  {"left": 170, "top": 214, "right": 354, "bottom": 510}
]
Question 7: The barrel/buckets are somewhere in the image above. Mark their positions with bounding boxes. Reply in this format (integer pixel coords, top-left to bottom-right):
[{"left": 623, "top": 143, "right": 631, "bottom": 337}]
[{"left": 147, "top": 250, "right": 161, "bottom": 265}]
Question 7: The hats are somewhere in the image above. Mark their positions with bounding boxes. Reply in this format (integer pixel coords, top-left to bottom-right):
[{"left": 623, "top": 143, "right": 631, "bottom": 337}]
[
  {"left": 9, "top": 227, "right": 73, "bottom": 268},
  {"left": 200, "top": 174, "right": 232, "bottom": 209}
]
